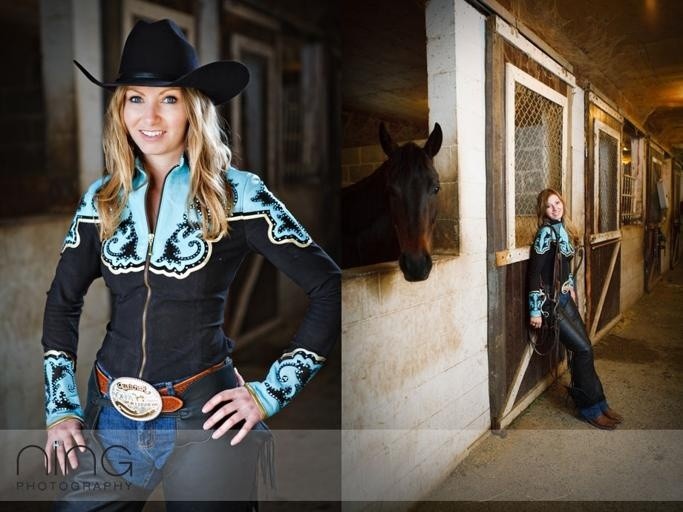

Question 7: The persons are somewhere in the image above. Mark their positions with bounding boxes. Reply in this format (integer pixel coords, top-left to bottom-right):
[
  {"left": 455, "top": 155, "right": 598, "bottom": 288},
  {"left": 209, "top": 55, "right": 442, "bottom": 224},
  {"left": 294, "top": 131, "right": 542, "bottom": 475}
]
[
  {"left": 527, "top": 186, "right": 624, "bottom": 430},
  {"left": 44, "top": 85, "right": 341, "bottom": 511}
]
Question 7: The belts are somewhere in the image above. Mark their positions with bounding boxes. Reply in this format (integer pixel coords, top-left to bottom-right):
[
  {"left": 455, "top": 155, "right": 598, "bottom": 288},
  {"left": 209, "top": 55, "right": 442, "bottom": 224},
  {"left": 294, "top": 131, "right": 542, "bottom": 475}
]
[{"left": 96, "top": 362, "right": 225, "bottom": 421}]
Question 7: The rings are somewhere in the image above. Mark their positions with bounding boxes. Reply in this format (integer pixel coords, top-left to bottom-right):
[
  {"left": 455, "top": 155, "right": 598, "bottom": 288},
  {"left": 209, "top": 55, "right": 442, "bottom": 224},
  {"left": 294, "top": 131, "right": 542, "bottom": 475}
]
[{"left": 51, "top": 441, "right": 61, "bottom": 451}]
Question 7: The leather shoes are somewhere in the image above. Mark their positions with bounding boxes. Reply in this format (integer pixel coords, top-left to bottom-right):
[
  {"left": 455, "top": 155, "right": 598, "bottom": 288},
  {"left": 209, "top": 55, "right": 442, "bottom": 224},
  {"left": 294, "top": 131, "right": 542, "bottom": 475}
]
[
  {"left": 601, "top": 399, "right": 623, "bottom": 424},
  {"left": 574, "top": 404, "right": 617, "bottom": 430}
]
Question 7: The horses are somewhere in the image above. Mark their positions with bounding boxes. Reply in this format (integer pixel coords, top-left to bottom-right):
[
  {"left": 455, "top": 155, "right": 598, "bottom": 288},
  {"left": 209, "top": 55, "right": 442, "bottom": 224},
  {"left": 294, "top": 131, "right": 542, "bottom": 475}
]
[{"left": 266, "top": 121, "right": 443, "bottom": 283}]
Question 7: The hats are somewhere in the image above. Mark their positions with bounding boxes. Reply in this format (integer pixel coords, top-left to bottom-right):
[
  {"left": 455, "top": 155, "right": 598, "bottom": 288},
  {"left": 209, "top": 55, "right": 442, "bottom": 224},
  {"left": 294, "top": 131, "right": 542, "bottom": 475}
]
[{"left": 72, "top": 19, "right": 252, "bottom": 107}]
